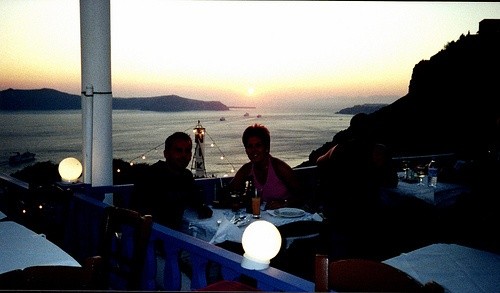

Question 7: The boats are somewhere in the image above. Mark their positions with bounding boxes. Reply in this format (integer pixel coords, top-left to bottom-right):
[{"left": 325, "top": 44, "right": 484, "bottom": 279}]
[
  {"left": 220, "top": 117, "right": 225, "bottom": 121},
  {"left": 257, "top": 114, "right": 261, "bottom": 118},
  {"left": 244, "top": 112, "right": 249, "bottom": 117}
]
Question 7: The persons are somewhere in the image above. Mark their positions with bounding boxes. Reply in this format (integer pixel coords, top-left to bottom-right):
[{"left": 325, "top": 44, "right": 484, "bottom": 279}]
[
  {"left": 132, "top": 132, "right": 213, "bottom": 264},
  {"left": 227, "top": 126, "right": 302, "bottom": 210},
  {"left": 316, "top": 113, "right": 397, "bottom": 188}
]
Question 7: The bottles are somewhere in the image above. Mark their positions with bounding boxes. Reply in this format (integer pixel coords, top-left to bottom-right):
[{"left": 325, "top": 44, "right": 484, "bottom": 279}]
[{"left": 403, "top": 156, "right": 438, "bottom": 189}]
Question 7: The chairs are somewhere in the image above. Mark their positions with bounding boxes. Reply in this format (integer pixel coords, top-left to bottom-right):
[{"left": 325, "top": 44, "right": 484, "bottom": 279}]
[
  {"left": 271, "top": 220, "right": 447, "bottom": 293},
  {"left": 75, "top": 206, "right": 153, "bottom": 293}
]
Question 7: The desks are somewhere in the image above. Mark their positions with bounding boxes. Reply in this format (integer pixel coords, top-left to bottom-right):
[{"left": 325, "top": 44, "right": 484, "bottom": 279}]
[
  {"left": 380, "top": 242, "right": 500, "bottom": 293},
  {"left": 395, "top": 171, "right": 482, "bottom": 237},
  {"left": 182, "top": 204, "right": 326, "bottom": 284}
]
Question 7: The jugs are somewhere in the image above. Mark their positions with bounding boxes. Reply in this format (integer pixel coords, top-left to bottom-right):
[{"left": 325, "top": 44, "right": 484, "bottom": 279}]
[{"left": 233, "top": 174, "right": 254, "bottom": 213}]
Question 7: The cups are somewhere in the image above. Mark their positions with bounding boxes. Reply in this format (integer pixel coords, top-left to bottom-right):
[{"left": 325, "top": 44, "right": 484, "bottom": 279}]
[
  {"left": 251, "top": 189, "right": 262, "bottom": 219},
  {"left": 419, "top": 175, "right": 426, "bottom": 186}
]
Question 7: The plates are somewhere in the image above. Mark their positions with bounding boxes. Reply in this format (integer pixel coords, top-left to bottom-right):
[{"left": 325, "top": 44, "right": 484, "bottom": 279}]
[
  {"left": 274, "top": 207, "right": 306, "bottom": 217},
  {"left": 216, "top": 216, "right": 250, "bottom": 228}
]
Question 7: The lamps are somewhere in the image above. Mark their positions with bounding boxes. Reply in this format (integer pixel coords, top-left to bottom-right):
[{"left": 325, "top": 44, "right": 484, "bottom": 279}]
[
  {"left": 58, "top": 157, "right": 83, "bottom": 184},
  {"left": 241, "top": 220, "right": 282, "bottom": 270}
]
